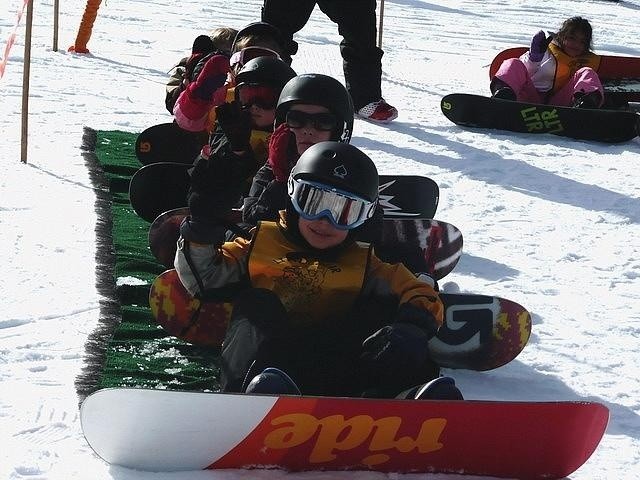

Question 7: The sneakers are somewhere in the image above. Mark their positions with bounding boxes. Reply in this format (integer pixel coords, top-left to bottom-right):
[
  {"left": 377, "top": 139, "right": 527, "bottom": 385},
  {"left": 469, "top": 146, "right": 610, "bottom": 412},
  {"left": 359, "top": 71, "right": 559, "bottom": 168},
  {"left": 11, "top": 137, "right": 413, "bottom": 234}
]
[
  {"left": 494, "top": 86, "right": 516, "bottom": 101},
  {"left": 574, "top": 95, "right": 597, "bottom": 111},
  {"left": 354, "top": 97, "right": 398, "bottom": 123}
]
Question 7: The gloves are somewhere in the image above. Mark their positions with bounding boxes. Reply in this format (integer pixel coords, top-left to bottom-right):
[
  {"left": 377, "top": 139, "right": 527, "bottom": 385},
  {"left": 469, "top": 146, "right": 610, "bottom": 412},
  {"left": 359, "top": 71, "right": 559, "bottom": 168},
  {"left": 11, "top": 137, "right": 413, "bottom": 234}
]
[
  {"left": 216, "top": 102, "right": 252, "bottom": 149},
  {"left": 362, "top": 304, "right": 436, "bottom": 377},
  {"left": 179, "top": 55, "right": 229, "bottom": 122},
  {"left": 529, "top": 30, "right": 553, "bottom": 63},
  {"left": 179, "top": 153, "right": 242, "bottom": 245}
]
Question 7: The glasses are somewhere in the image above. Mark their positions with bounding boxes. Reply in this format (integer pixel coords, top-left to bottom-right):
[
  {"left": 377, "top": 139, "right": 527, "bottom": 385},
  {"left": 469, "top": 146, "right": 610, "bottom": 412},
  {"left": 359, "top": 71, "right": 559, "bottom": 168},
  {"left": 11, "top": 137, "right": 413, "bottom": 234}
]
[{"left": 290, "top": 181, "right": 372, "bottom": 230}]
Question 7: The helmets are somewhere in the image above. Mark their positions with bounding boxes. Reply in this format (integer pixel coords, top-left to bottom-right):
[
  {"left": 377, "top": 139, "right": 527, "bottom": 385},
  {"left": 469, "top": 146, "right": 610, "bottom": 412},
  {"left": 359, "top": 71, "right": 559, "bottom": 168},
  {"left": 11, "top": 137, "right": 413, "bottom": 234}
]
[
  {"left": 229, "top": 21, "right": 354, "bottom": 144},
  {"left": 287, "top": 141, "right": 380, "bottom": 229}
]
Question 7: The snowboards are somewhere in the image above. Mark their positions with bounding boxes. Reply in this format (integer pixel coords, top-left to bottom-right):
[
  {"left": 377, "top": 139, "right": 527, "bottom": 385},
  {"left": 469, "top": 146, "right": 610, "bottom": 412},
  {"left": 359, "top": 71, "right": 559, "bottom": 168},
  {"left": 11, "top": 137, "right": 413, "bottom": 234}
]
[
  {"left": 134, "top": 122, "right": 274, "bottom": 166},
  {"left": 81, "top": 387, "right": 609, "bottom": 480},
  {"left": 148, "top": 266, "right": 532, "bottom": 370},
  {"left": 147, "top": 207, "right": 464, "bottom": 282},
  {"left": 129, "top": 162, "right": 442, "bottom": 225},
  {"left": 490, "top": 49, "right": 640, "bottom": 83},
  {"left": 441, "top": 93, "right": 639, "bottom": 145}
]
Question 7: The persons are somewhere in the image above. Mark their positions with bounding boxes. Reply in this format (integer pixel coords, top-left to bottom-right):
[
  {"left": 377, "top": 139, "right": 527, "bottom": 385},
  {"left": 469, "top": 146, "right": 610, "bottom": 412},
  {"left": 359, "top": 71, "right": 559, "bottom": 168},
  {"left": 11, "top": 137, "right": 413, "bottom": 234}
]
[
  {"left": 489, "top": 16, "right": 607, "bottom": 111},
  {"left": 259, "top": 0, "right": 398, "bottom": 124}
]
